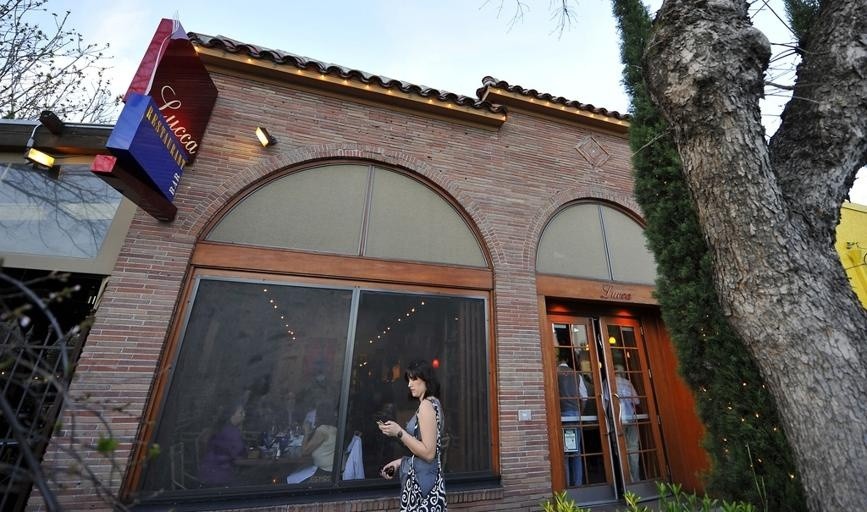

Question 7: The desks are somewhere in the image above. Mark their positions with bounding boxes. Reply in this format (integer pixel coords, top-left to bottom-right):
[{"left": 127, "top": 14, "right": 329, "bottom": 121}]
[{"left": 232, "top": 457, "right": 313, "bottom": 481}]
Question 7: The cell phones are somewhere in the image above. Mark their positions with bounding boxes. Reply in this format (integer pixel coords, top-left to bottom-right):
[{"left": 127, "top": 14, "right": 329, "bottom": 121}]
[{"left": 376, "top": 419, "right": 385, "bottom": 425}]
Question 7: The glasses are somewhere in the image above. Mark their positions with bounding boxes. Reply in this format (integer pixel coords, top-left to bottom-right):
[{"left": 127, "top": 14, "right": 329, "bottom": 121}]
[{"left": 378, "top": 465, "right": 395, "bottom": 477}]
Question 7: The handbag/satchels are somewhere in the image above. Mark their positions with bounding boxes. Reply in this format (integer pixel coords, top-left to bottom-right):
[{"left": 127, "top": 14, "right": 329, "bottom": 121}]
[{"left": 262, "top": 429, "right": 291, "bottom": 454}]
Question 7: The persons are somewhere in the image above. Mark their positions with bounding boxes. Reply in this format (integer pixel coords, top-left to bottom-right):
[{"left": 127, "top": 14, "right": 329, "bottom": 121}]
[
  {"left": 555, "top": 349, "right": 589, "bottom": 487},
  {"left": 194, "top": 382, "right": 337, "bottom": 488},
  {"left": 602, "top": 364, "right": 641, "bottom": 484},
  {"left": 375, "top": 357, "right": 449, "bottom": 511}
]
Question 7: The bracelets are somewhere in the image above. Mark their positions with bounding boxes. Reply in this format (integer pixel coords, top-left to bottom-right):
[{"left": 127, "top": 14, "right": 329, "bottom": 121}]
[{"left": 397, "top": 429, "right": 403, "bottom": 439}]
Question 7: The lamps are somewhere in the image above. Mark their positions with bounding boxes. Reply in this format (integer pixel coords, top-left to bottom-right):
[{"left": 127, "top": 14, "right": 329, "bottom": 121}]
[
  {"left": 24, "top": 147, "right": 61, "bottom": 180},
  {"left": 255, "top": 127, "right": 277, "bottom": 148}
]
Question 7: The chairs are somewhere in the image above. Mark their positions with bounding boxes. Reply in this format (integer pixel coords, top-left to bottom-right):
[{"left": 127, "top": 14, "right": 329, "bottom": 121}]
[{"left": 169, "top": 428, "right": 210, "bottom": 491}]
